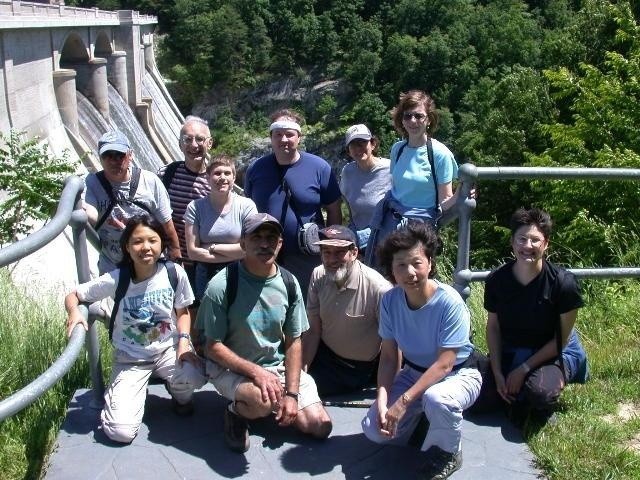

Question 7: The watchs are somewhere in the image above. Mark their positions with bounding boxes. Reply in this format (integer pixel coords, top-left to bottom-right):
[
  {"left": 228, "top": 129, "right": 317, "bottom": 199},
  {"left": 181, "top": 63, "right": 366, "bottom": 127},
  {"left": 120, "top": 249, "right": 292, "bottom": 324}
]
[{"left": 209, "top": 243, "right": 217, "bottom": 253}]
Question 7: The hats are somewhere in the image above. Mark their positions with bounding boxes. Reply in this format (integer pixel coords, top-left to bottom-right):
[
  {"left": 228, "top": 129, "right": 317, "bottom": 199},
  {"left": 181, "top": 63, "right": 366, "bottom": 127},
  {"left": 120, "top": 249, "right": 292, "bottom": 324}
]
[
  {"left": 98, "top": 131, "right": 129, "bottom": 155},
  {"left": 242, "top": 213, "right": 285, "bottom": 235},
  {"left": 344, "top": 124, "right": 372, "bottom": 147},
  {"left": 312, "top": 225, "right": 357, "bottom": 247}
]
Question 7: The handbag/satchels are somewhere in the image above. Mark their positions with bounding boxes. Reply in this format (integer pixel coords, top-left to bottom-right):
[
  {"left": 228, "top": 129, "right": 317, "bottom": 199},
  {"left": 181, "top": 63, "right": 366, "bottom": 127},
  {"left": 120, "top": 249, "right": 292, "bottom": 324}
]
[{"left": 298, "top": 222, "right": 325, "bottom": 256}]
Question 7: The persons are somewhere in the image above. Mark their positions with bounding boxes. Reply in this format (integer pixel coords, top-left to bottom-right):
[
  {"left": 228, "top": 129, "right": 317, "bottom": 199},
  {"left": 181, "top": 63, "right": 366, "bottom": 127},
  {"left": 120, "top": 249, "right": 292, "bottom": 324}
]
[
  {"left": 371, "top": 91, "right": 458, "bottom": 284},
  {"left": 301, "top": 224, "right": 395, "bottom": 395},
  {"left": 361, "top": 224, "right": 484, "bottom": 480},
  {"left": 184, "top": 155, "right": 259, "bottom": 306},
  {"left": 193, "top": 212, "right": 332, "bottom": 454},
  {"left": 468, "top": 208, "right": 591, "bottom": 425},
  {"left": 243, "top": 110, "right": 342, "bottom": 301},
  {"left": 64, "top": 214, "right": 207, "bottom": 444},
  {"left": 339, "top": 123, "right": 392, "bottom": 264},
  {"left": 81, "top": 129, "right": 183, "bottom": 280},
  {"left": 156, "top": 114, "right": 213, "bottom": 359}
]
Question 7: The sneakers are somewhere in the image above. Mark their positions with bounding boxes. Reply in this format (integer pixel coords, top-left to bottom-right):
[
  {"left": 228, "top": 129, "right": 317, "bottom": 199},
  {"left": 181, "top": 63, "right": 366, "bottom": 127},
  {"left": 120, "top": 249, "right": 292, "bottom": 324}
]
[
  {"left": 224, "top": 401, "right": 250, "bottom": 453},
  {"left": 415, "top": 450, "right": 462, "bottom": 480},
  {"left": 165, "top": 382, "right": 195, "bottom": 417}
]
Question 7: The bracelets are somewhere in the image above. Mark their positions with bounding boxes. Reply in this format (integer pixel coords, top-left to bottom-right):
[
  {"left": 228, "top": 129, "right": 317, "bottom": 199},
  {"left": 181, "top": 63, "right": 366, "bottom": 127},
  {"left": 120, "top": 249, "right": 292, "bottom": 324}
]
[
  {"left": 401, "top": 393, "right": 413, "bottom": 405},
  {"left": 283, "top": 390, "right": 301, "bottom": 403},
  {"left": 519, "top": 362, "right": 532, "bottom": 374},
  {"left": 178, "top": 333, "right": 189, "bottom": 340}
]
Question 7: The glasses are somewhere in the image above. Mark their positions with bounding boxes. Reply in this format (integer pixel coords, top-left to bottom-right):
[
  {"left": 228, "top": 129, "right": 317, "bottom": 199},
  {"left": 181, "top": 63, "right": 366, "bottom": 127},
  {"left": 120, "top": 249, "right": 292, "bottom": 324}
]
[
  {"left": 511, "top": 235, "right": 545, "bottom": 249},
  {"left": 179, "top": 136, "right": 210, "bottom": 145},
  {"left": 401, "top": 111, "right": 429, "bottom": 120}
]
